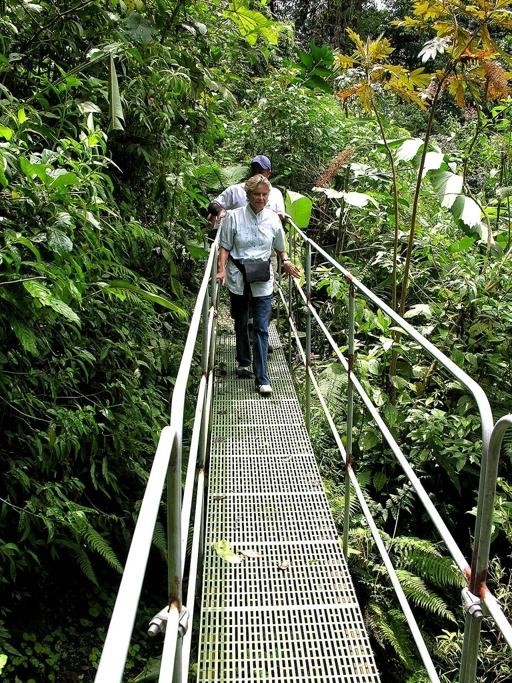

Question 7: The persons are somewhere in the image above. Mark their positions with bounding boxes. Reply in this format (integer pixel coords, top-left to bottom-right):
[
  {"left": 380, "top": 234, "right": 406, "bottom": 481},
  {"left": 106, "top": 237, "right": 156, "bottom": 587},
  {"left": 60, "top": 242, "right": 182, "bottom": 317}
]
[
  {"left": 216, "top": 174, "right": 302, "bottom": 393},
  {"left": 208, "top": 155, "right": 286, "bottom": 353}
]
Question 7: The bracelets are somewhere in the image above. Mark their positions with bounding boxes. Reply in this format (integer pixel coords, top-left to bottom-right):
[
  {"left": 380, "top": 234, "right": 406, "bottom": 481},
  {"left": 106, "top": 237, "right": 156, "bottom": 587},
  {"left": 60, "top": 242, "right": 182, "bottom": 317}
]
[{"left": 281, "top": 256, "right": 290, "bottom": 263}]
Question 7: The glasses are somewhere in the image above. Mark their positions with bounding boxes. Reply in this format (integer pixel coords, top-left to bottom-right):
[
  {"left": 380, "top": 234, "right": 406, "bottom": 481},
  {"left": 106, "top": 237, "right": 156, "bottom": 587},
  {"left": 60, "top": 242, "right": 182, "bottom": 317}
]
[{"left": 252, "top": 170, "right": 265, "bottom": 174}]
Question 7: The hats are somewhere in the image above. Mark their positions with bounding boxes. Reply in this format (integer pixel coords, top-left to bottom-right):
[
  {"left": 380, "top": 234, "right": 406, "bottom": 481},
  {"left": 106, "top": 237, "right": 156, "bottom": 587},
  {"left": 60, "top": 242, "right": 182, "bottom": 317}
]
[{"left": 250, "top": 154, "right": 271, "bottom": 171}]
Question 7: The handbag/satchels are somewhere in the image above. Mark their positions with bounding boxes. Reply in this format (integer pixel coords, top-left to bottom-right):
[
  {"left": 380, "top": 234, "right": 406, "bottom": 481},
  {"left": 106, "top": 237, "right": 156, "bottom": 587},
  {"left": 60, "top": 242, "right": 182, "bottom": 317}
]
[{"left": 233, "top": 258, "right": 272, "bottom": 284}]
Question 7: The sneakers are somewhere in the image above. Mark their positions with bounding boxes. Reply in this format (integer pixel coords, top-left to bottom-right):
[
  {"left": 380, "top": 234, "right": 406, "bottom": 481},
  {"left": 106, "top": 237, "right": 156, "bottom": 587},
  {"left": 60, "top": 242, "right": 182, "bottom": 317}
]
[
  {"left": 238, "top": 365, "right": 253, "bottom": 376},
  {"left": 255, "top": 384, "right": 273, "bottom": 395}
]
